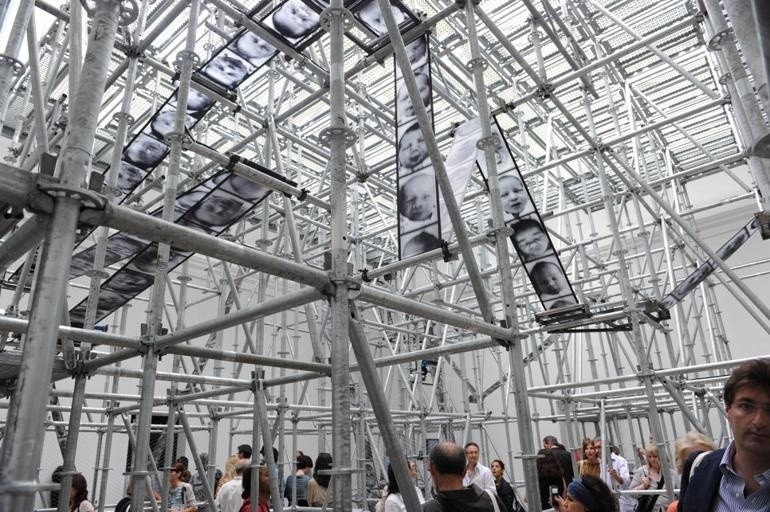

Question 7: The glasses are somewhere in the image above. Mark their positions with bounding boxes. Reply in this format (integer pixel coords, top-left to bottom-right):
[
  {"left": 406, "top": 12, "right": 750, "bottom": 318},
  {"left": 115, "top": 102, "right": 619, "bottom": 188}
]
[{"left": 732, "top": 399, "right": 770, "bottom": 417}]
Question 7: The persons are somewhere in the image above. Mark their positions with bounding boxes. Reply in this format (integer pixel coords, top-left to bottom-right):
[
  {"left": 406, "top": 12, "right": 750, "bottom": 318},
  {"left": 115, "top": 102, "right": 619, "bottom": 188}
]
[
  {"left": 102, "top": 27, "right": 279, "bottom": 195},
  {"left": 52, "top": 465, "right": 95, "bottom": 510},
  {"left": 272, "top": 0, "right": 321, "bottom": 41},
  {"left": 478, "top": 129, "right": 509, "bottom": 174},
  {"left": 11, "top": 224, "right": 141, "bottom": 292},
  {"left": 674, "top": 356, "right": 769, "bottom": 510},
  {"left": 50, "top": 430, "right": 722, "bottom": 510},
  {"left": 117, "top": 453, "right": 223, "bottom": 508},
  {"left": 527, "top": 260, "right": 569, "bottom": 298},
  {"left": 548, "top": 299, "right": 576, "bottom": 314},
  {"left": 524, "top": 436, "right": 714, "bottom": 510},
  {"left": 497, "top": 174, "right": 529, "bottom": 215},
  {"left": 357, "top": 1, "right": 407, "bottom": 36},
  {"left": 395, "top": 35, "right": 439, "bottom": 259},
  {"left": 507, "top": 217, "right": 554, "bottom": 263},
  {"left": 376, "top": 441, "right": 522, "bottom": 509},
  {"left": 216, "top": 445, "right": 333, "bottom": 510},
  {"left": 8, "top": 173, "right": 270, "bottom": 330}
]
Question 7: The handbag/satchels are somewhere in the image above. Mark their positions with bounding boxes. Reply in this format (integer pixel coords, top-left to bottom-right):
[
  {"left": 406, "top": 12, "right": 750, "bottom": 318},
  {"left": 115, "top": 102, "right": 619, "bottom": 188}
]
[{"left": 633, "top": 495, "right": 650, "bottom": 512}]
